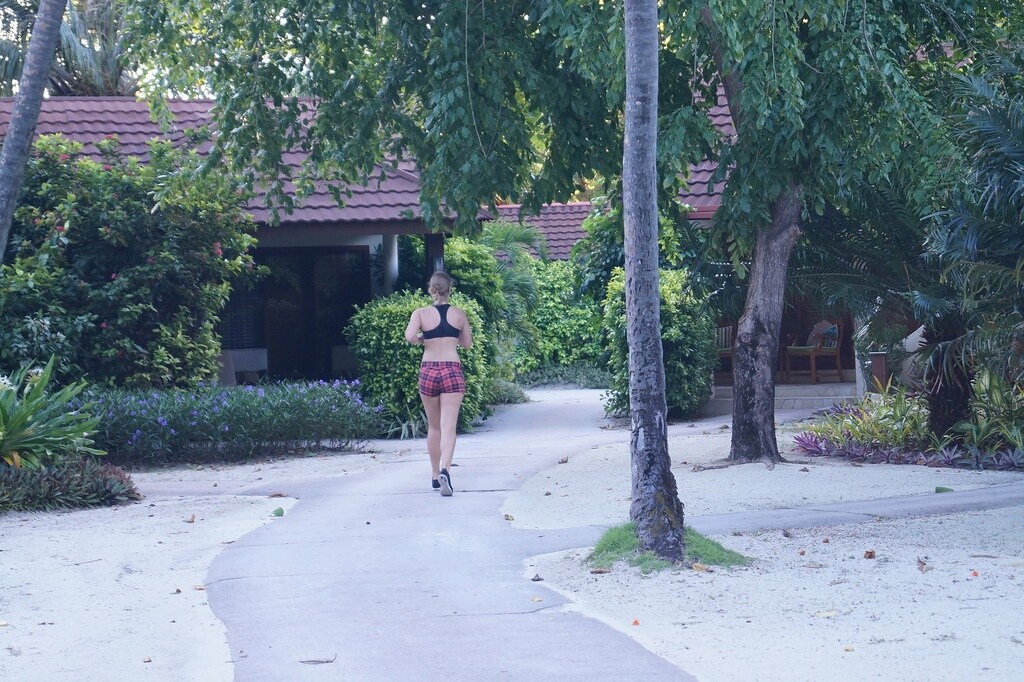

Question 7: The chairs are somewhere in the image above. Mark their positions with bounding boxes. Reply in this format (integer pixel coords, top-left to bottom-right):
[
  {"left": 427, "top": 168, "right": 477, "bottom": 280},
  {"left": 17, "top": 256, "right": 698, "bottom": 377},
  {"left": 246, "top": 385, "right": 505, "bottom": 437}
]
[
  {"left": 712, "top": 322, "right": 737, "bottom": 357},
  {"left": 786, "top": 318, "right": 844, "bottom": 384}
]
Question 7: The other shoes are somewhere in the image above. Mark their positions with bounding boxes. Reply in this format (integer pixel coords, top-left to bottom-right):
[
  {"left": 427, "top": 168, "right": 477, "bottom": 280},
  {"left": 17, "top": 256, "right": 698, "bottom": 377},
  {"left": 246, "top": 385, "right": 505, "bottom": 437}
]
[
  {"left": 437, "top": 468, "right": 453, "bottom": 496},
  {"left": 431, "top": 478, "right": 441, "bottom": 490}
]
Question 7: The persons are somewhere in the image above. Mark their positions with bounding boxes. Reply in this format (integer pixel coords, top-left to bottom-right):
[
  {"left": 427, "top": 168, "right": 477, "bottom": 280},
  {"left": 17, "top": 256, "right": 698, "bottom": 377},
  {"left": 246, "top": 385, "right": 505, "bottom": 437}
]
[{"left": 405, "top": 272, "right": 472, "bottom": 496}]
